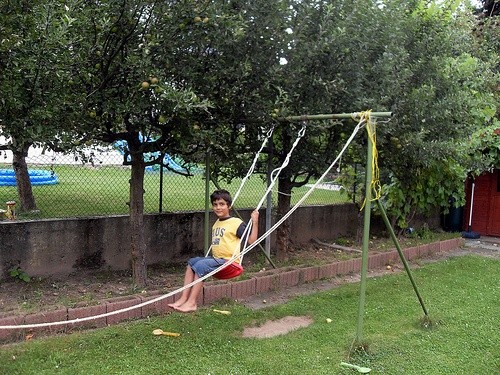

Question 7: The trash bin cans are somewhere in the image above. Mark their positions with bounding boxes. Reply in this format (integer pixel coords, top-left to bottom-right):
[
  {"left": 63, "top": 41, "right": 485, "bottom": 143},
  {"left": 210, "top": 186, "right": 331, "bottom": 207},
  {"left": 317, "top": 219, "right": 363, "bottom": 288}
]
[
  {"left": 464, "top": 168, "right": 500, "bottom": 237},
  {"left": 434, "top": 193, "right": 465, "bottom": 233}
]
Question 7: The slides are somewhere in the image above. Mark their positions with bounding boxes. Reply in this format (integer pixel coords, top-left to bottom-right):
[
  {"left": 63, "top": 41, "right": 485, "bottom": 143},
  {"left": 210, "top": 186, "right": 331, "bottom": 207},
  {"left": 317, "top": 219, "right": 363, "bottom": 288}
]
[{"left": 114, "top": 136, "right": 195, "bottom": 171}]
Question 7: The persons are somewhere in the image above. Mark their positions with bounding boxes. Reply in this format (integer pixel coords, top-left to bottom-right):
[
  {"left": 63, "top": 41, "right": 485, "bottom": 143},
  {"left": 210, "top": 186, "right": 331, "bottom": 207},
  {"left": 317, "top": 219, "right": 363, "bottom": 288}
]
[{"left": 167, "top": 188, "right": 260, "bottom": 313}]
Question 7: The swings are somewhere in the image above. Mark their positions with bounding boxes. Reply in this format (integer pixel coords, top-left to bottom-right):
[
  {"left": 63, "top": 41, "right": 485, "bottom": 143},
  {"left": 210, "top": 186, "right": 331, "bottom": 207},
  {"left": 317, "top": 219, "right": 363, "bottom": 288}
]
[{"left": 203, "top": 122, "right": 307, "bottom": 279}]
